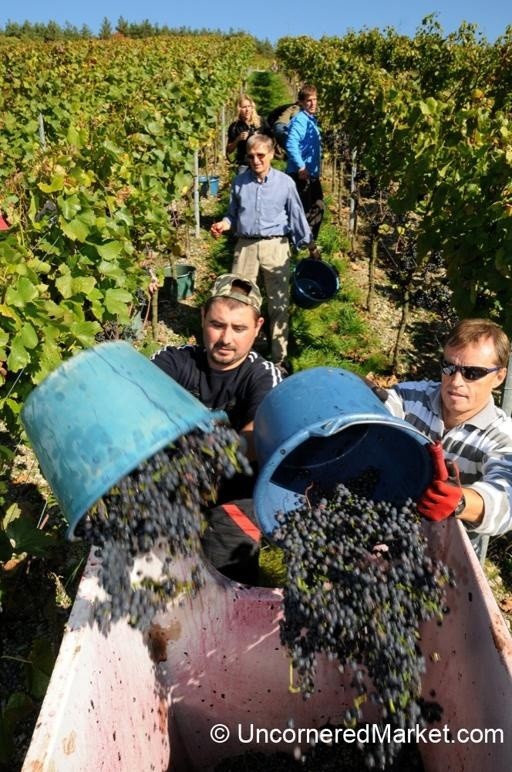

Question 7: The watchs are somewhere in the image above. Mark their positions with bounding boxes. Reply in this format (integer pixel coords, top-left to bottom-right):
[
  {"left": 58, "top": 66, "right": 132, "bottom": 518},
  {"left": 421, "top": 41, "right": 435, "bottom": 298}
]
[{"left": 452, "top": 493, "right": 466, "bottom": 516}]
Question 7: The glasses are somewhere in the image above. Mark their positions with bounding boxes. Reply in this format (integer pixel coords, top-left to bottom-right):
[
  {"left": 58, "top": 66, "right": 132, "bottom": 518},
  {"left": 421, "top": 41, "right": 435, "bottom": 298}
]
[
  {"left": 437, "top": 360, "right": 502, "bottom": 382},
  {"left": 247, "top": 152, "right": 269, "bottom": 159}
]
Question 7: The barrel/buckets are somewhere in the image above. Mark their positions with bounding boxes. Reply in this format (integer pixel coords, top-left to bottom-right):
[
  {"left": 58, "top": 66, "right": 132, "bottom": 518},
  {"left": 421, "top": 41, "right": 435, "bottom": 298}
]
[
  {"left": 160, "top": 264, "right": 197, "bottom": 300},
  {"left": 250, "top": 364, "right": 451, "bottom": 537},
  {"left": 198, "top": 175, "right": 221, "bottom": 195},
  {"left": 17, "top": 337, "right": 231, "bottom": 544},
  {"left": 289, "top": 256, "right": 342, "bottom": 311}
]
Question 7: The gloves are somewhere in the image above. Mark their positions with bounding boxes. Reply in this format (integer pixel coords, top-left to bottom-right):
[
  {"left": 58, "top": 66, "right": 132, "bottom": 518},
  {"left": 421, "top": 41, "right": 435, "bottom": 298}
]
[{"left": 416, "top": 459, "right": 464, "bottom": 523}]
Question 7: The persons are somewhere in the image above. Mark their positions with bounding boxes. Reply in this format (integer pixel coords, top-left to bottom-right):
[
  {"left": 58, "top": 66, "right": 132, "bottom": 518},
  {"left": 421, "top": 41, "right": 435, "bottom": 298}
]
[
  {"left": 210, "top": 135, "right": 322, "bottom": 377},
  {"left": 149, "top": 275, "right": 283, "bottom": 468},
  {"left": 226, "top": 94, "right": 286, "bottom": 176},
  {"left": 372, "top": 319, "right": 512, "bottom": 567},
  {"left": 267, "top": 99, "right": 302, "bottom": 147},
  {"left": 285, "top": 85, "right": 323, "bottom": 242}
]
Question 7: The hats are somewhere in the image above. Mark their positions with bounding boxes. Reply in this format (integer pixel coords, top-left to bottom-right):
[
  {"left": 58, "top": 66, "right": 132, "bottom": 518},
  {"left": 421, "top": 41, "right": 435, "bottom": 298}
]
[{"left": 203, "top": 273, "right": 263, "bottom": 314}]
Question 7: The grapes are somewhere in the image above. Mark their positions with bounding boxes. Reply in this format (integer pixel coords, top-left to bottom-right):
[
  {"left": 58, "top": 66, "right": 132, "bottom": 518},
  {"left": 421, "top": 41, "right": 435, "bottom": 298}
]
[{"left": 58, "top": 424, "right": 458, "bottom": 772}]
